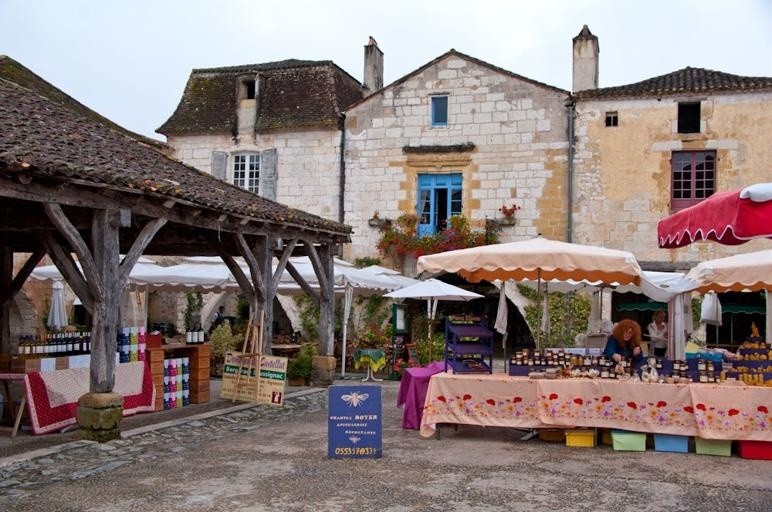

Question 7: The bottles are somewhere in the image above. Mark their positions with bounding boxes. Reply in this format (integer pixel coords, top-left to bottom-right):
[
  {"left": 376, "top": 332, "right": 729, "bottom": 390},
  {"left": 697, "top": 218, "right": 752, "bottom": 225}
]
[
  {"left": 185, "top": 322, "right": 204, "bottom": 343},
  {"left": 18, "top": 332, "right": 91, "bottom": 360}
]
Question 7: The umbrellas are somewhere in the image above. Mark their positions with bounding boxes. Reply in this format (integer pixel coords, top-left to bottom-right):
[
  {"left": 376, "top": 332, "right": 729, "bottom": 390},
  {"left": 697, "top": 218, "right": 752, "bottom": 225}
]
[
  {"left": 657, "top": 182, "right": 772, "bottom": 346},
  {"left": 382, "top": 278, "right": 484, "bottom": 365},
  {"left": 416, "top": 233, "right": 640, "bottom": 351}
]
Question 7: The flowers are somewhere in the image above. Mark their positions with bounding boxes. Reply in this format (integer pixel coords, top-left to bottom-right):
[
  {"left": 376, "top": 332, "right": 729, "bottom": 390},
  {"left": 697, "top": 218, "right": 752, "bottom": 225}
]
[
  {"left": 375, "top": 203, "right": 521, "bottom": 259},
  {"left": 287, "top": 282, "right": 592, "bottom": 387}
]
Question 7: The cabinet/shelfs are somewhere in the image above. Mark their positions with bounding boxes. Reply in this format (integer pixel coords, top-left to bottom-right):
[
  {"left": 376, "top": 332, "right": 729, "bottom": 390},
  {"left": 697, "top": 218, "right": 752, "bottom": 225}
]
[
  {"left": 397, "top": 368, "right": 443, "bottom": 430},
  {"left": 0, "top": 361, "right": 156, "bottom": 438},
  {"left": 417, "top": 370, "right": 771, "bottom": 440}
]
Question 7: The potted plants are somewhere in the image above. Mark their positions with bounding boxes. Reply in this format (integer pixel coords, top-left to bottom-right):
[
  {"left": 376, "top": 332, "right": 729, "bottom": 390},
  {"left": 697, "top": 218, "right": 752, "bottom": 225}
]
[{"left": 206, "top": 317, "right": 244, "bottom": 378}]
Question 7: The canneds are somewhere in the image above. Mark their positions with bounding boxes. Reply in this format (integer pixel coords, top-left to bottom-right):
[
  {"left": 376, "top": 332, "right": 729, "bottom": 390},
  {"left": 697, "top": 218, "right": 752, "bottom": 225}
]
[{"left": 511, "top": 349, "right": 715, "bottom": 383}]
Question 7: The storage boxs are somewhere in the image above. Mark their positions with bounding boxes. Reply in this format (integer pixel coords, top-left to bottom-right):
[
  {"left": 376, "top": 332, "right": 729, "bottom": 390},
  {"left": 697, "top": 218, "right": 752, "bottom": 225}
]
[
  {"left": 11, "top": 352, "right": 119, "bottom": 372},
  {"left": 566, "top": 430, "right": 595, "bottom": 447},
  {"left": 653, "top": 434, "right": 690, "bottom": 452},
  {"left": 738, "top": 440, "right": 771, "bottom": 459},
  {"left": 149, "top": 343, "right": 213, "bottom": 411},
  {"left": 695, "top": 436, "right": 732, "bottom": 456},
  {"left": 610, "top": 429, "right": 647, "bottom": 451}
]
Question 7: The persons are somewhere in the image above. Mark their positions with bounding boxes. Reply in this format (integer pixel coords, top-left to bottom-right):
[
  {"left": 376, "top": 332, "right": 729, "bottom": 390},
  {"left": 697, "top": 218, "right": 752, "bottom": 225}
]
[
  {"left": 605, "top": 319, "right": 642, "bottom": 363},
  {"left": 648, "top": 308, "right": 669, "bottom": 359}
]
